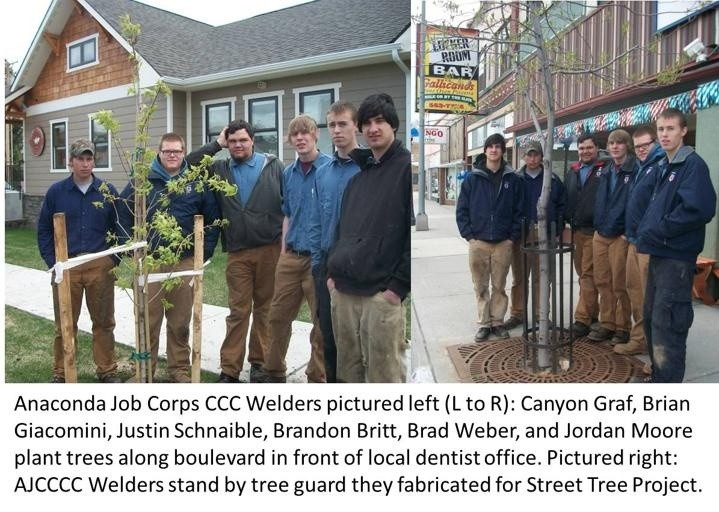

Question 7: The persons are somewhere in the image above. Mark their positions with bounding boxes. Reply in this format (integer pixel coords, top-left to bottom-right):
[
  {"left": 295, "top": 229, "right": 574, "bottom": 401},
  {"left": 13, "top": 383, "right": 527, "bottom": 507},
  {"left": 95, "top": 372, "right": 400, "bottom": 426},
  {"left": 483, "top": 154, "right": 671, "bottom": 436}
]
[
  {"left": 501, "top": 140, "right": 566, "bottom": 331},
  {"left": 185, "top": 119, "right": 285, "bottom": 383},
  {"left": 262, "top": 114, "right": 333, "bottom": 383},
  {"left": 564, "top": 131, "right": 611, "bottom": 339},
  {"left": 455, "top": 133, "right": 528, "bottom": 342},
  {"left": 307, "top": 99, "right": 365, "bottom": 383},
  {"left": 36, "top": 136, "right": 121, "bottom": 383},
  {"left": 324, "top": 93, "right": 411, "bottom": 383},
  {"left": 613, "top": 123, "right": 660, "bottom": 376},
  {"left": 118, "top": 132, "right": 221, "bottom": 383},
  {"left": 635, "top": 107, "right": 718, "bottom": 383},
  {"left": 585, "top": 128, "right": 638, "bottom": 345}
]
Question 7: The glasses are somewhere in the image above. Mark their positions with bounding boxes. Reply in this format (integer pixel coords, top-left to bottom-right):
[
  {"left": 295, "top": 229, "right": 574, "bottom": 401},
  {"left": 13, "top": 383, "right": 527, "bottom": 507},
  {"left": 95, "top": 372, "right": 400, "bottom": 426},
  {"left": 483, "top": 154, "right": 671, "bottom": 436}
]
[
  {"left": 159, "top": 149, "right": 183, "bottom": 157},
  {"left": 632, "top": 137, "right": 657, "bottom": 151}
]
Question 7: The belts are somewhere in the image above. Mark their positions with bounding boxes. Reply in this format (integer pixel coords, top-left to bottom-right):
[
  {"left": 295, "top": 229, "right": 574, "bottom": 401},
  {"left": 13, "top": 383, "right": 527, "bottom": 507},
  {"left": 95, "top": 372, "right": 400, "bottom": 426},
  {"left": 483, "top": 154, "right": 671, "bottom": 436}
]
[{"left": 287, "top": 248, "right": 311, "bottom": 256}]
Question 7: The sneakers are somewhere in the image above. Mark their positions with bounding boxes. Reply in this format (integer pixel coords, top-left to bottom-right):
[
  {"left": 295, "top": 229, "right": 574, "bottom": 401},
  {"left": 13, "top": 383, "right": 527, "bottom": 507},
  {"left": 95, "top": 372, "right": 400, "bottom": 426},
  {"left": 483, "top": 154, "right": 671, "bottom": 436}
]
[
  {"left": 614, "top": 339, "right": 649, "bottom": 353},
  {"left": 537, "top": 320, "right": 552, "bottom": 327},
  {"left": 492, "top": 326, "right": 509, "bottom": 339},
  {"left": 611, "top": 331, "right": 630, "bottom": 346},
  {"left": 216, "top": 373, "right": 239, "bottom": 383},
  {"left": 587, "top": 327, "right": 616, "bottom": 342},
  {"left": 48, "top": 375, "right": 65, "bottom": 383},
  {"left": 475, "top": 328, "right": 490, "bottom": 342},
  {"left": 505, "top": 317, "right": 523, "bottom": 330},
  {"left": 103, "top": 374, "right": 123, "bottom": 383},
  {"left": 172, "top": 375, "right": 192, "bottom": 383},
  {"left": 569, "top": 319, "right": 598, "bottom": 338}
]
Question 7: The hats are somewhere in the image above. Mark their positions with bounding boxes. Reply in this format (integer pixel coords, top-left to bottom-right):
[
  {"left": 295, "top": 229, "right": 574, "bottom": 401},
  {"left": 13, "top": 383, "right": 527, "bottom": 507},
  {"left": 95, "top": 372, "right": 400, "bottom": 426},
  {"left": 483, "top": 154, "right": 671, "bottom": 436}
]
[
  {"left": 71, "top": 139, "right": 95, "bottom": 157},
  {"left": 524, "top": 140, "right": 542, "bottom": 155}
]
